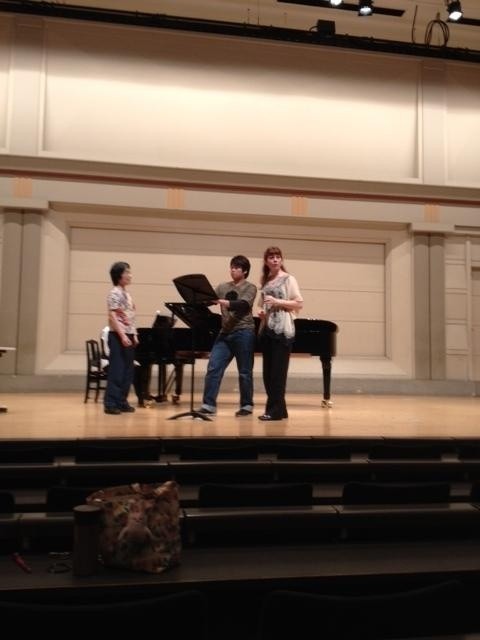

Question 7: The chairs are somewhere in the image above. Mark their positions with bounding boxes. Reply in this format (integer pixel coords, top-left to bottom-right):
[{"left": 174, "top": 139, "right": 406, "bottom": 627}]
[{"left": 84, "top": 336, "right": 108, "bottom": 403}]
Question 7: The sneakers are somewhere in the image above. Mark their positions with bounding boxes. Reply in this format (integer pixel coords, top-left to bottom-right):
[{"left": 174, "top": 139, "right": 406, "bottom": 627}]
[
  {"left": 104, "top": 404, "right": 136, "bottom": 414},
  {"left": 258, "top": 411, "right": 288, "bottom": 421},
  {"left": 192, "top": 408, "right": 216, "bottom": 420},
  {"left": 235, "top": 408, "right": 253, "bottom": 417}
]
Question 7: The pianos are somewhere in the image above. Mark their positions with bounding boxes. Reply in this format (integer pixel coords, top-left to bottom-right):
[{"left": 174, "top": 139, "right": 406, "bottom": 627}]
[{"left": 136, "top": 302, "right": 337, "bottom": 408}]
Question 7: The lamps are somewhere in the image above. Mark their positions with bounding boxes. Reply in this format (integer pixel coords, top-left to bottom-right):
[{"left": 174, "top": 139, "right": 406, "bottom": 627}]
[{"left": 329, "top": 0, "right": 464, "bottom": 21}]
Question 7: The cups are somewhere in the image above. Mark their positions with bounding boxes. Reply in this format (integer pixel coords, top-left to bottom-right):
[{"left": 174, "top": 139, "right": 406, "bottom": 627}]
[{"left": 72, "top": 504, "right": 105, "bottom": 576}]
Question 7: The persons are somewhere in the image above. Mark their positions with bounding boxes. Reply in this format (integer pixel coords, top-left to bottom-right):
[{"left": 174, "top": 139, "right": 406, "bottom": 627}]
[
  {"left": 255, "top": 243, "right": 307, "bottom": 422},
  {"left": 101, "top": 260, "right": 140, "bottom": 417},
  {"left": 195, "top": 253, "right": 258, "bottom": 418}
]
